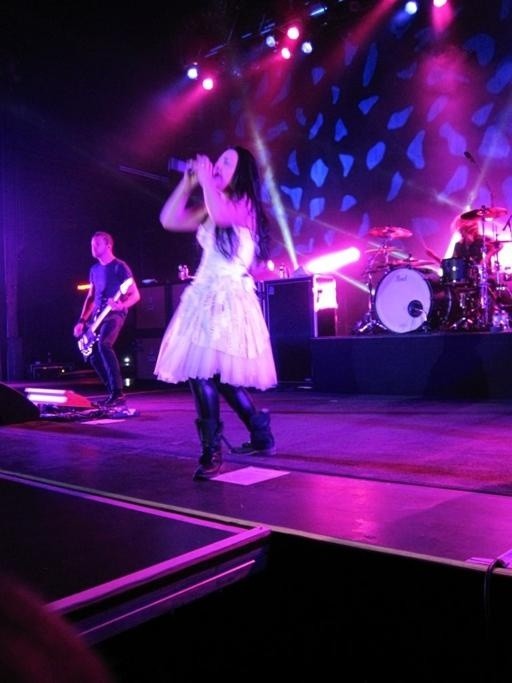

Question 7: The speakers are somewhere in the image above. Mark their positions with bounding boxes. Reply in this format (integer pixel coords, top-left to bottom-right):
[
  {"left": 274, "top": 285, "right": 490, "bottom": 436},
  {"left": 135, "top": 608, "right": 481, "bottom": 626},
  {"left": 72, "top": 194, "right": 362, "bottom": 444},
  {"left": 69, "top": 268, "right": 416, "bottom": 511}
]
[{"left": 0, "top": 383, "right": 40, "bottom": 427}]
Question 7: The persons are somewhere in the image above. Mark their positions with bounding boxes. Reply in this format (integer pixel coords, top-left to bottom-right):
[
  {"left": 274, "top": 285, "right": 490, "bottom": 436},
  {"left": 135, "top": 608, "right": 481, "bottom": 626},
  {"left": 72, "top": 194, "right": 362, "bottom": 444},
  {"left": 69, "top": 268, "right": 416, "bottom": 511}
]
[
  {"left": 74, "top": 231, "right": 141, "bottom": 407},
  {"left": 453, "top": 223, "right": 504, "bottom": 263},
  {"left": 153, "top": 145, "right": 278, "bottom": 480}
]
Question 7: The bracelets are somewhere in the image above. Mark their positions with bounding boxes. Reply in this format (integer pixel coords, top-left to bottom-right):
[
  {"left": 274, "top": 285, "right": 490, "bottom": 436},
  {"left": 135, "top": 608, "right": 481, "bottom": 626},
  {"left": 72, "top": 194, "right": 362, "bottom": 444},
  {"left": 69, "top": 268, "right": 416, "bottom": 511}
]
[{"left": 78, "top": 318, "right": 85, "bottom": 325}]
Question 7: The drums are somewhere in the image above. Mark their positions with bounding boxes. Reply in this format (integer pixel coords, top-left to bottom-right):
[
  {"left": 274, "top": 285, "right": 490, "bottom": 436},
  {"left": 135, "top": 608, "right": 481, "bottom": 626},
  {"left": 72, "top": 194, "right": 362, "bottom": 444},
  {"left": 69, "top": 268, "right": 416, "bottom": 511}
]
[
  {"left": 369, "top": 259, "right": 411, "bottom": 290},
  {"left": 373, "top": 266, "right": 452, "bottom": 334},
  {"left": 465, "top": 263, "right": 491, "bottom": 283},
  {"left": 438, "top": 285, "right": 495, "bottom": 331}
]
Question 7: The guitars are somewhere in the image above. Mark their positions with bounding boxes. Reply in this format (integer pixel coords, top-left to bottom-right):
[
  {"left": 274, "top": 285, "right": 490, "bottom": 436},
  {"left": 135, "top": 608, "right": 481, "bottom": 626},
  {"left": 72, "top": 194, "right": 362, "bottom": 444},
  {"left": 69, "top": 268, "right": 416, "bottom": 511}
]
[{"left": 76, "top": 277, "right": 134, "bottom": 358}]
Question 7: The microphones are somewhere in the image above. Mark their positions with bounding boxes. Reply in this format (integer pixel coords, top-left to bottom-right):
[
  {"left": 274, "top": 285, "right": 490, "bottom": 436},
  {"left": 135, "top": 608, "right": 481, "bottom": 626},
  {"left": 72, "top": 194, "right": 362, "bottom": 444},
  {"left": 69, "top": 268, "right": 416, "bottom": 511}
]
[
  {"left": 502, "top": 216, "right": 512, "bottom": 230},
  {"left": 463, "top": 150, "right": 476, "bottom": 165},
  {"left": 168, "top": 158, "right": 196, "bottom": 177}
]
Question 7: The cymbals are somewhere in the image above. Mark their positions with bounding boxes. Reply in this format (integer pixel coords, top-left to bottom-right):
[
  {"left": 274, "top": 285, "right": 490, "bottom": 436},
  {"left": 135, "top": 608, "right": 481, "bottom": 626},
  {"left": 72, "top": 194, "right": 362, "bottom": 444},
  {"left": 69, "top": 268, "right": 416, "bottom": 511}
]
[
  {"left": 461, "top": 207, "right": 508, "bottom": 220},
  {"left": 367, "top": 224, "right": 413, "bottom": 239}
]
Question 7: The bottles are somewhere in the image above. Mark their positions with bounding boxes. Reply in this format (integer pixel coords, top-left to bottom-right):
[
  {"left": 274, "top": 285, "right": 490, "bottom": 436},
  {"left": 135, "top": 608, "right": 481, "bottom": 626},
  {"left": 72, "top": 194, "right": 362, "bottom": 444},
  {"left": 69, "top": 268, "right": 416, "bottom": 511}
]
[{"left": 279, "top": 265, "right": 286, "bottom": 279}]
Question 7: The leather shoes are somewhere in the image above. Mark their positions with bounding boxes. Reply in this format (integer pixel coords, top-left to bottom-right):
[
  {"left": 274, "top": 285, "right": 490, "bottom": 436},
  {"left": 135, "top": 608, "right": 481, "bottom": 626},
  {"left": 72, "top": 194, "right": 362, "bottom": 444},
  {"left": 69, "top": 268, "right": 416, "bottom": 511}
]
[{"left": 97, "top": 393, "right": 126, "bottom": 407}]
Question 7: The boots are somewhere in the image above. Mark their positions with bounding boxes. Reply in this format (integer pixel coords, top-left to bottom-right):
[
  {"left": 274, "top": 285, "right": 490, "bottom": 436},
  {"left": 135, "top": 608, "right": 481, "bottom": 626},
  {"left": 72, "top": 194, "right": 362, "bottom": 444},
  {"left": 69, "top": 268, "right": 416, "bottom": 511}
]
[
  {"left": 192, "top": 418, "right": 223, "bottom": 478},
  {"left": 228, "top": 410, "right": 276, "bottom": 457}
]
